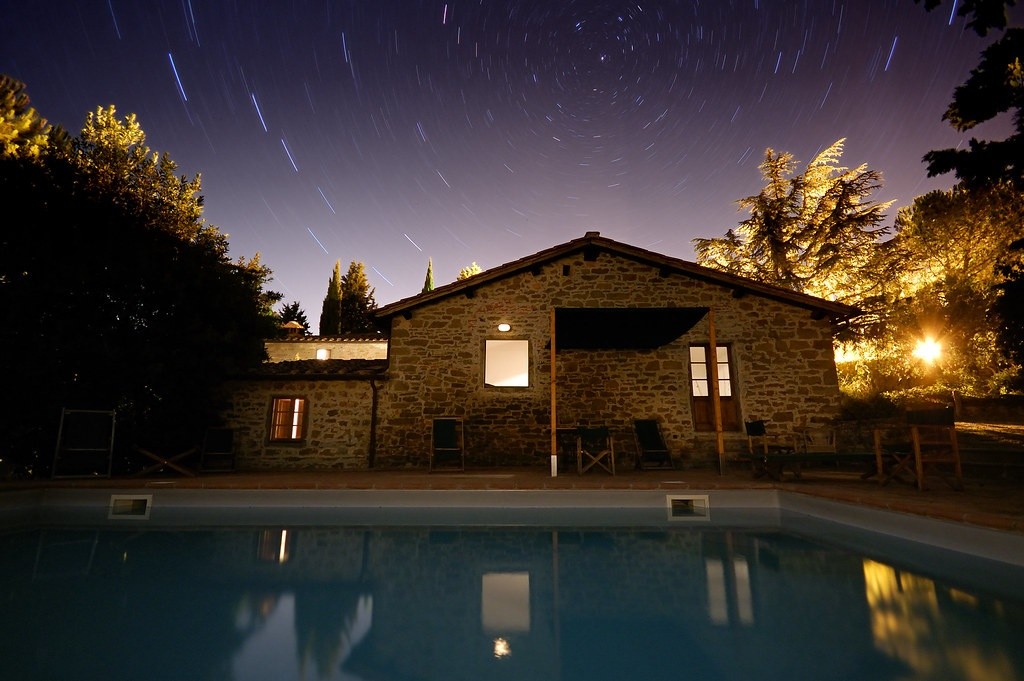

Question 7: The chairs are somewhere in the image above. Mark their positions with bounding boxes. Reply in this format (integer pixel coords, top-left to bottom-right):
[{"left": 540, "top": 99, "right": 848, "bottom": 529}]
[
  {"left": 428, "top": 416, "right": 465, "bottom": 473},
  {"left": 803, "top": 426, "right": 836, "bottom": 453},
  {"left": 200, "top": 426, "right": 242, "bottom": 476},
  {"left": 630, "top": 419, "right": 673, "bottom": 470}
]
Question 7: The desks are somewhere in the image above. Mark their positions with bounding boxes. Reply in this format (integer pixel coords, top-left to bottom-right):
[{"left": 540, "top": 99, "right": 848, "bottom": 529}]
[
  {"left": 745, "top": 432, "right": 798, "bottom": 455},
  {"left": 872, "top": 421, "right": 961, "bottom": 488},
  {"left": 546, "top": 425, "right": 620, "bottom": 476}
]
